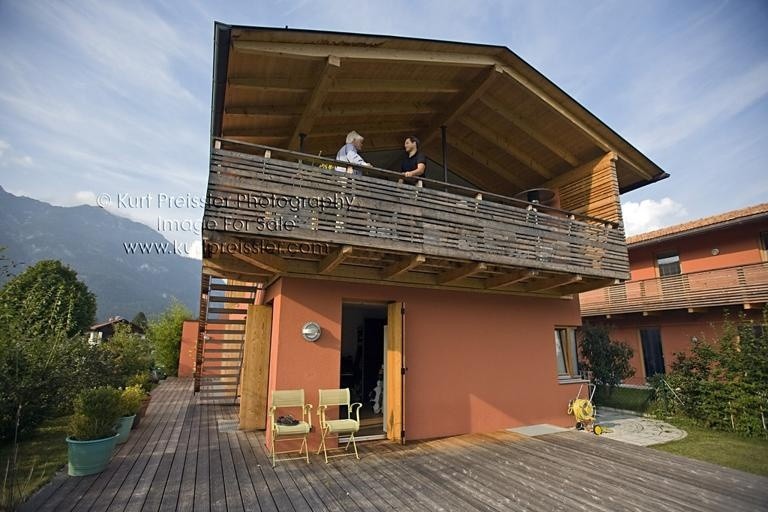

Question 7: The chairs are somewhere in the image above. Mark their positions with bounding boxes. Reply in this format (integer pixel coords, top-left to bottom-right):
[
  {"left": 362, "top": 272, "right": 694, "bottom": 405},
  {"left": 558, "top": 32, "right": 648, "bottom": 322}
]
[
  {"left": 267, "top": 389, "right": 313, "bottom": 468},
  {"left": 316, "top": 387, "right": 363, "bottom": 464}
]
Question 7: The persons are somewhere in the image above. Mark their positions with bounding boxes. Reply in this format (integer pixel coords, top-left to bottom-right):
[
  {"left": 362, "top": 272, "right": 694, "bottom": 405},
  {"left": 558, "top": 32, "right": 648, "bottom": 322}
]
[
  {"left": 399, "top": 134, "right": 428, "bottom": 186},
  {"left": 335, "top": 130, "right": 373, "bottom": 175}
]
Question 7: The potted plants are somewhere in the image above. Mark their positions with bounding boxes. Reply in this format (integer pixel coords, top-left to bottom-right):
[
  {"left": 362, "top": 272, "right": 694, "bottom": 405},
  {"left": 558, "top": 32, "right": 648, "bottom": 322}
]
[{"left": 64, "top": 384, "right": 152, "bottom": 477}]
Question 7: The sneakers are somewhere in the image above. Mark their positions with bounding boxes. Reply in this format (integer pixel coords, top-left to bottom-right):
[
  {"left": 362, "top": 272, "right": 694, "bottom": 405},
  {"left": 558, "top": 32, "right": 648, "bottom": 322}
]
[{"left": 277, "top": 415, "right": 299, "bottom": 425}]
